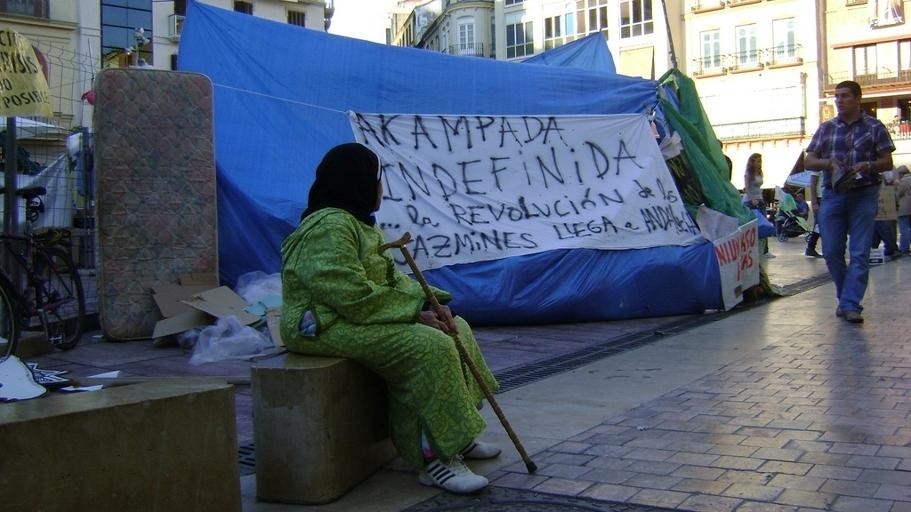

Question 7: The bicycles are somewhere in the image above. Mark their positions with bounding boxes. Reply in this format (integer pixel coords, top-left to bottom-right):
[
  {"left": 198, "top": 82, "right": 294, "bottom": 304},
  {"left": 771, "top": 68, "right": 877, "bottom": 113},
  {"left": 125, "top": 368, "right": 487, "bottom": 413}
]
[{"left": 0, "top": 184, "right": 85, "bottom": 363}]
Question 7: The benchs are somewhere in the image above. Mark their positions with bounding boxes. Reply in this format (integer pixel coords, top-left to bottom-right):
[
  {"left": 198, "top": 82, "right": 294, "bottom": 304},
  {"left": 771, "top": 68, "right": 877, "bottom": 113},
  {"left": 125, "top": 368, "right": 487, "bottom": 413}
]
[
  {"left": 0, "top": 381, "right": 245, "bottom": 512},
  {"left": 250, "top": 352, "right": 398, "bottom": 505}
]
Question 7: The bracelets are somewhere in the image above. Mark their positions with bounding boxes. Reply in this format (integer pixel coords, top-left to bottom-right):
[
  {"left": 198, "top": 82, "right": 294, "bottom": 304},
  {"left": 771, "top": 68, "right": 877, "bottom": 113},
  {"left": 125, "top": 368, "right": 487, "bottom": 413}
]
[{"left": 866, "top": 160, "right": 874, "bottom": 174}]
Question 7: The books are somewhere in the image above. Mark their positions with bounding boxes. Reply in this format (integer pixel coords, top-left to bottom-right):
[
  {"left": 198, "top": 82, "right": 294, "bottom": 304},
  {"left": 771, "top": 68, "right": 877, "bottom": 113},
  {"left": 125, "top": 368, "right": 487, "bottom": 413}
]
[{"left": 832, "top": 164, "right": 865, "bottom": 194}]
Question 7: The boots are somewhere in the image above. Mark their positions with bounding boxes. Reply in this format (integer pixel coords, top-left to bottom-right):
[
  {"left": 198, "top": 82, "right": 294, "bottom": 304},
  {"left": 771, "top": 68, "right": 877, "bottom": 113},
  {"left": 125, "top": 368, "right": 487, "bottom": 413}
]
[{"left": 804, "top": 231, "right": 823, "bottom": 258}]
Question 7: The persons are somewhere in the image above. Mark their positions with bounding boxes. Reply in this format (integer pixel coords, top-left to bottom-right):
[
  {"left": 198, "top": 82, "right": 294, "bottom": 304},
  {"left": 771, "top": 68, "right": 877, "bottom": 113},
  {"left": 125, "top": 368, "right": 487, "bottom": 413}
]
[
  {"left": 870, "top": 163, "right": 911, "bottom": 263},
  {"left": 742, "top": 153, "right": 777, "bottom": 259},
  {"left": 891, "top": 114, "right": 901, "bottom": 135},
  {"left": 803, "top": 168, "right": 826, "bottom": 259},
  {"left": 802, "top": 81, "right": 896, "bottom": 324},
  {"left": 789, "top": 191, "right": 810, "bottom": 226},
  {"left": 717, "top": 139, "right": 733, "bottom": 181},
  {"left": 274, "top": 142, "right": 502, "bottom": 494},
  {"left": 777, "top": 209, "right": 790, "bottom": 237}
]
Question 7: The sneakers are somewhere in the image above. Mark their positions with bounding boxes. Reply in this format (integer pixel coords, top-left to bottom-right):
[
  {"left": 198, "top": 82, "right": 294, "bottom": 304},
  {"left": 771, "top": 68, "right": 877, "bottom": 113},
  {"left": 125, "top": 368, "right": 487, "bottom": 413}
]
[
  {"left": 459, "top": 439, "right": 502, "bottom": 460},
  {"left": 764, "top": 253, "right": 776, "bottom": 258},
  {"left": 417, "top": 453, "right": 489, "bottom": 494},
  {"left": 836, "top": 307, "right": 864, "bottom": 323}
]
